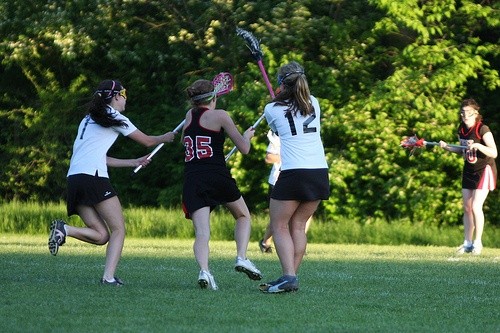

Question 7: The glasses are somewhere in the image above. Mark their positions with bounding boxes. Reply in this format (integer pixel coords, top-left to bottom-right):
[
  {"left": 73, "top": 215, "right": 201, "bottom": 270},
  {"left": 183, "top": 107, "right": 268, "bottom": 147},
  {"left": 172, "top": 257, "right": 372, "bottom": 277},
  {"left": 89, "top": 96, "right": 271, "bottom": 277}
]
[{"left": 461, "top": 110, "right": 478, "bottom": 116}]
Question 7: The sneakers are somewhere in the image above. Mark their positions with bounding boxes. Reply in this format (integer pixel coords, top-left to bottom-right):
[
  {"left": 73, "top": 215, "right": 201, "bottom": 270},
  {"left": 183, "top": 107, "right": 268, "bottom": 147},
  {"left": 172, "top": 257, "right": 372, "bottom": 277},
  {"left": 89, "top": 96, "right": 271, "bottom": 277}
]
[
  {"left": 101, "top": 277, "right": 123, "bottom": 285},
  {"left": 263, "top": 278, "right": 299, "bottom": 292},
  {"left": 473, "top": 243, "right": 482, "bottom": 255},
  {"left": 259, "top": 281, "right": 276, "bottom": 290},
  {"left": 235, "top": 256, "right": 261, "bottom": 280},
  {"left": 260, "top": 239, "right": 272, "bottom": 253},
  {"left": 198, "top": 270, "right": 218, "bottom": 291},
  {"left": 48, "top": 220, "right": 66, "bottom": 256},
  {"left": 456, "top": 245, "right": 472, "bottom": 252}
]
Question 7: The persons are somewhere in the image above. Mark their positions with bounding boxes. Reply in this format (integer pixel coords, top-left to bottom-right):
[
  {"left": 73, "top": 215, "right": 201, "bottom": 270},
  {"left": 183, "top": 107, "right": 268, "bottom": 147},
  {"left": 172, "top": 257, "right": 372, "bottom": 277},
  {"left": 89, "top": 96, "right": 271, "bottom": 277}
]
[
  {"left": 180, "top": 79, "right": 263, "bottom": 290},
  {"left": 48, "top": 80, "right": 178, "bottom": 286},
  {"left": 259, "top": 130, "right": 311, "bottom": 254},
  {"left": 258, "top": 62, "right": 330, "bottom": 293},
  {"left": 440, "top": 99, "right": 498, "bottom": 255}
]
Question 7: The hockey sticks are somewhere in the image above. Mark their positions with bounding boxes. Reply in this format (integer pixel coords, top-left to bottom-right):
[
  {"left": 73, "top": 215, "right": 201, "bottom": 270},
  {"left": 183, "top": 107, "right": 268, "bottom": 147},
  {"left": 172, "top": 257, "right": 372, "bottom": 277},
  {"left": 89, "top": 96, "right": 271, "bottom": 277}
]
[
  {"left": 399, "top": 134, "right": 470, "bottom": 150},
  {"left": 225, "top": 87, "right": 282, "bottom": 164},
  {"left": 133, "top": 72, "right": 233, "bottom": 174},
  {"left": 235, "top": 28, "right": 276, "bottom": 100}
]
